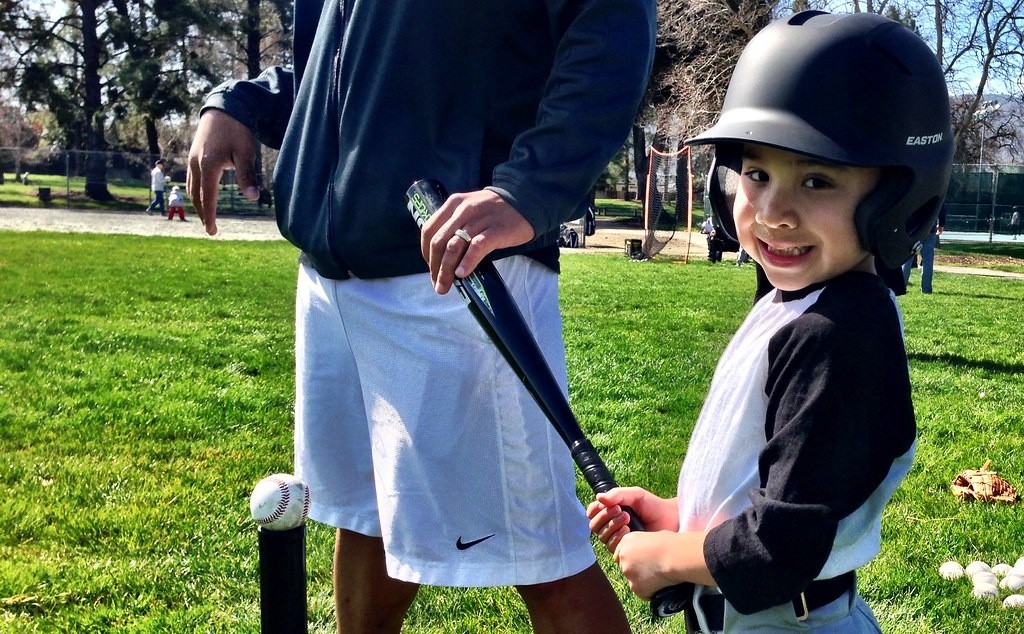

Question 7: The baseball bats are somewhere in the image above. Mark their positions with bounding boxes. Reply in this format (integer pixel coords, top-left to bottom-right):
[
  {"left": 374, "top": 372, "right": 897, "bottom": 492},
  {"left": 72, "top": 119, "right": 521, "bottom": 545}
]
[{"left": 399, "top": 174, "right": 690, "bottom": 617}]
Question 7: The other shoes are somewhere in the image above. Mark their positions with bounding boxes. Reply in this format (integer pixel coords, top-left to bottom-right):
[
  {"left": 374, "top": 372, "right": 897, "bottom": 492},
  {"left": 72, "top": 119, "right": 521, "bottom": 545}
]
[
  {"left": 179, "top": 219, "right": 188, "bottom": 222},
  {"left": 161, "top": 213, "right": 167, "bottom": 216},
  {"left": 167, "top": 218, "right": 172, "bottom": 220},
  {"left": 145, "top": 209, "right": 152, "bottom": 216}
]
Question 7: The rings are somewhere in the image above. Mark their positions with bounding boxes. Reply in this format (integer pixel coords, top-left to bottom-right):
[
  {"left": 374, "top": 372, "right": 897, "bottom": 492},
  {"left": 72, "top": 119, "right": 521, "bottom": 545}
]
[{"left": 454, "top": 228, "right": 470, "bottom": 243}]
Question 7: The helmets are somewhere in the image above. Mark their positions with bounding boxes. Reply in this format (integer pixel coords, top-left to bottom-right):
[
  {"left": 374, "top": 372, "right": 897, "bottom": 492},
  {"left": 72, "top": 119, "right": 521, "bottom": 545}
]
[{"left": 684, "top": 8, "right": 954, "bottom": 296}]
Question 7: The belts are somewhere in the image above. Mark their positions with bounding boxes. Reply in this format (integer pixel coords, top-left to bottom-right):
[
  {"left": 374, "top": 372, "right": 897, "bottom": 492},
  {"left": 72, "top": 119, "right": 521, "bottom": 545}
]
[{"left": 684, "top": 571, "right": 853, "bottom": 634}]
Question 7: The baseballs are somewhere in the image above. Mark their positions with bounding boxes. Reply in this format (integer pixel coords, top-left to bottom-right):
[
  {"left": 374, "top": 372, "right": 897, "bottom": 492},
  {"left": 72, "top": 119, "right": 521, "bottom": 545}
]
[{"left": 247, "top": 474, "right": 312, "bottom": 531}]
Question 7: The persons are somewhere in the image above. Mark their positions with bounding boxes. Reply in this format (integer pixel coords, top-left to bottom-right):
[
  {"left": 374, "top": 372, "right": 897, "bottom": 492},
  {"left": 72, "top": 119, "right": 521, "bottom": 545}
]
[
  {"left": 893, "top": 196, "right": 947, "bottom": 293},
  {"left": 183, "top": 1, "right": 656, "bottom": 633},
  {"left": 582, "top": 10, "right": 951, "bottom": 634},
  {"left": 145, "top": 159, "right": 170, "bottom": 217},
  {"left": 1008, "top": 205, "right": 1020, "bottom": 240},
  {"left": 167, "top": 186, "right": 185, "bottom": 221}
]
